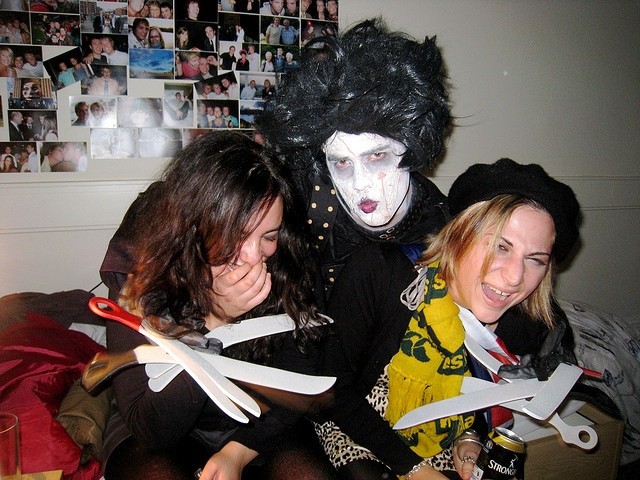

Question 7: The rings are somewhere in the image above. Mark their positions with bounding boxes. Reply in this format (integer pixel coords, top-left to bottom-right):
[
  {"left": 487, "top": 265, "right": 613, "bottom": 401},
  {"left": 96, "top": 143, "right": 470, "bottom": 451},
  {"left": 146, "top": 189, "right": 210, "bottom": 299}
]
[{"left": 462, "top": 457, "right": 477, "bottom": 463}]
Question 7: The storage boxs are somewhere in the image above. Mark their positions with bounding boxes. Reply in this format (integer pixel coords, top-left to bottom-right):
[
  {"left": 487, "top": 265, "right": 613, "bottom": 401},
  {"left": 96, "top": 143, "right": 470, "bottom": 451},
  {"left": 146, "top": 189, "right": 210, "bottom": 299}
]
[{"left": 522, "top": 400, "right": 625, "bottom": 480}]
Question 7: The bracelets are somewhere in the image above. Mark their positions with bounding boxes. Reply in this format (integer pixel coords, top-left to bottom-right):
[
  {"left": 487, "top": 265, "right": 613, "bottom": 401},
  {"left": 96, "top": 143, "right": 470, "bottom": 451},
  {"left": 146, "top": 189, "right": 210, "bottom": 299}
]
[
  {"left": 402, "top": 458, "right": 433, "bottom": 477},
  {"left": 455, "top": 437, "right": 485, "bottom": 446}
]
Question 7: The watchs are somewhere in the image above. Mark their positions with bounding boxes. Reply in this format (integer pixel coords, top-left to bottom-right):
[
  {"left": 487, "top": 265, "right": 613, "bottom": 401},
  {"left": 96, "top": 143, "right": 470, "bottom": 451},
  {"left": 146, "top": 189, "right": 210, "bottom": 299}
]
[{"left": 464, "top": 427, "right": 479, "bottom": 437}]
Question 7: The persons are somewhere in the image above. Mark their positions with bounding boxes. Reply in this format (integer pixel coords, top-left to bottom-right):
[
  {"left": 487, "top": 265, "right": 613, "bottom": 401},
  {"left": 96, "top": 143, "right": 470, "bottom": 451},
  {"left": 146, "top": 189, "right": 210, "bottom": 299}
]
[
  {"left": 41, "top": 145, "right": 63, "bottom": 173},
  {"left": 63, "top": 142, "right": 87, "bottom": 173},
  {"left": 0, "top": 20, "right": 30, "bottom": 44},
  {"left": 326, "top": 26, "right": 335, "bottom": 38},
  {"left": 184, "top": 0, "right": 200, "bottom": 21},
  {"left": 301, "top": 24, "right": 316, "bottom": 47},
  {"left": 284, "top": 0, "right": 299, "bottom": 17},
  {"left": 261, "top": 79, "right": 277, "bottom": 101},
  {"left": 221, "top": 77, "right": 240, "bottom": 100},
  {"left": 90, "top": 102, "right": 107, "bottom": 128},
  {"left": 209, "top": 106, "right": 233, "bottom": 130},
  {"left": 8, "top": 111, "right": 25, "bottom": 141},
  {"left": 207, "top": 106, "right": 214, "bottom": 129},
  {"left": 221, "top": 0, "right": 236, "bottom": 12},
  {"left": 34, "top": 115, "right": 47, "bottom": 141},
  {"left": 282, "top": 19, "right": 300, "bottom": 46},
  {"left": 201, "top": 26, "right": 219, "bottom": 52},
  {"left": 149, "top": 1, "right": 161, "bottom": 18},
  {"left": 236, "top": 50, "right": 249, "bottom": 72},
  {"left": 175, "top": 26, "right": 191, "bottom": 50},
  {"left": 273, "top": 48, "right": 286, "bottom": 74},
  {"left": 101, "top": 36, "right": 129, "bottom": 66},
  {"left": 0, "top": 47, "right": 16, "bottom": 77},
  {"left": 138, "top": 128, "right": 183, "bottom": 158},
  {"left": 321, "top": 28, "right": 328, "bottom": 36},
  {"left": 88, "top": 67, "right": 120, "bottom": 96},
  {"left": 105, "top": 137, "right": 121, "bottom": 158},
  {"left": 266, "top": 17, "right": 284, "bottom": 46},
  {"left": 127, "top": 98, "right": 163, "bottom": 128},
  {"left": 284, "top": 52, "right": 300, "bottom": 75},
  {"left": 149, "top": 26, "right": 165, "bottom": 49},
  {"left": 94, "top": 11, "right": 124, "bottom": 33},
  {"left": 84, "top": 38, "right": 108, "bottom": 78},
  {"left": 251, "top": 131, "right": 266, "bottom": 145},
  {"left": 231, "top": 25, "right": 246, "bottom": 41},
  {"left": 13, "top": 82, "right": 56, "bottom": 110},
  {"left": 25, "top": 115, "right": 40, "bottom": 142},
  {"left": 217, "top": 3, "right": 222, "bottom": 11},
  {"left": 260, "top": 0, "right": 285, "bottom": 16},
  {"left": 30, "top": 0, "right": 67, "bottom": 12},
  {"left": 0, "top": 0, "right": 27, "bottom": 12},
  {"left": 384, "top": 158, "right": 583, "bottom": 478},
  {"left": 262, "top": 51, "right": 274, "bottom": 73},
  {"left": 70, "top": 102, "right": 91, "bottom": 126},
  {"left": 240, "top": 80, "right": 257, "bottom": 101},
  {"left": 196, "top": 102, "right": 209, "bottom": 128},
  {"left": 102, "top": 132, "right": 338, "bottom": 480},
  {"left": 207, "top": 83, "right": 228, "bottom": 101},
  {"left": 222, "top": 106, "right": 238, "bottom": 128},
  {"left": 301, "top": 0, "right": 313, "bottom": 20},
  {"left": 70, "top": 57, "right": 91, "bottom": 88},
  {"left": 196, "top": 82, "right": 212, "bottom": 101},
  {"left": 160, "top": 2, "right": 173, "bottom": 19},
  {"left": 81, "top": 78, "right": 91, "bottom": 95},
  {"left": 14, "top": 55, "right": 31, "bottom": 79},
  {"left": 0, "top": 143, "right": 37, "bottom": 174},
  {"left": 314, "top": 0, "right": 328, "bottom": 20},
  {"left": 164, "top": 89, "right": 193, "bottom": 121},
  {"left": 127, "top": 0, "right": 145, "bottom": 17},
  {"left": 116, "top": 129, "right": 136, "bottom": 158},
  {"left": 32, "top": 15, "right": 80, "bottom": 46},
  {"left": 175, "top": 46, "right": 201, "bottom": 81},
  {"left": 325, "top": 0, "right": 338, "bottom": 21},
  {"left": 25, "top": 53, "right": 43, "bottom": 78},
  {"left": 220, "top": 46, "right": 236, "bottom": 71},
  {"left": 242, "top": 0, "right": 259, "bottom": 13},
  {"left": 199, "top": 55, "right": 219, "bottom": 81},
  {"left": 57, "top": 62, "right": 76, "bottom": 92},
  {"left": 45, "top": 115, "right": 57, "bottom": 142},
  {"left": 191, "top": 129, "right": 210, "bottom": 140},
  {"left": 128, "top": 18, "right": 149, "bottom": 49},
  {"left": 246, "top": 44, "right": 260, "bottom": 73},
  {"left": 250, "top": 25, "right": 453, "bottom": 480},
  {"left": 134, "top": 4, "right": 150, "bottom": 17}
]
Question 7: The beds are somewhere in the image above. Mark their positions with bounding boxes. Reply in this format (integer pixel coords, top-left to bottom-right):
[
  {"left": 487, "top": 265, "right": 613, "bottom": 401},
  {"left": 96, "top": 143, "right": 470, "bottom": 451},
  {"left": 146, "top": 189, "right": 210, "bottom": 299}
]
[{"left": 1, "top": 293, "right": 640, "bottom": 480}]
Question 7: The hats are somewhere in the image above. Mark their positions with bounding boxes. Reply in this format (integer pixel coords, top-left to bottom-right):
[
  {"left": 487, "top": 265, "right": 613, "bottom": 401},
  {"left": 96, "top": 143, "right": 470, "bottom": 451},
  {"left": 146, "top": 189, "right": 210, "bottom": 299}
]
[{"left": 443, "top": 156, "right": 584, "bottom": 276}]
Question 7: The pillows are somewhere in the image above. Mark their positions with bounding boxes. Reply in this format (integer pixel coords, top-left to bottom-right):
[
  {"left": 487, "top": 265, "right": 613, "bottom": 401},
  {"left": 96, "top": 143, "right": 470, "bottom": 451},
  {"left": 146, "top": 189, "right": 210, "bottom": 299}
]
[{"left": 1, "top": 312, "right": 110, "bottom": 480}]
